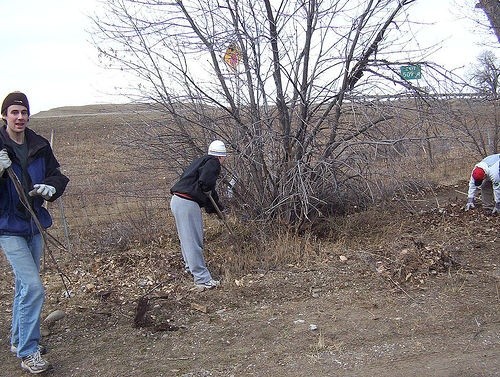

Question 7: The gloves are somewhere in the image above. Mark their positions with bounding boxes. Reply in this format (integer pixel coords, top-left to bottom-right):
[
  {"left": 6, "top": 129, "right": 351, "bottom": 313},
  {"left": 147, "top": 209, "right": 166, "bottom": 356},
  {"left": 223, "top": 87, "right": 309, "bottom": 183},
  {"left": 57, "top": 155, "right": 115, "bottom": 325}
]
[
  {"left": 465, "top": 198, "right": 476, "bottom": 211},
  {"left": 492, "top": 203, "right": 500, "bottom": 215},
  {"left": 0, "top": 148, "right": 12, "bottom": 177},
  {"left": 28, "top": 183, "right": 56, "bottom": 200}
]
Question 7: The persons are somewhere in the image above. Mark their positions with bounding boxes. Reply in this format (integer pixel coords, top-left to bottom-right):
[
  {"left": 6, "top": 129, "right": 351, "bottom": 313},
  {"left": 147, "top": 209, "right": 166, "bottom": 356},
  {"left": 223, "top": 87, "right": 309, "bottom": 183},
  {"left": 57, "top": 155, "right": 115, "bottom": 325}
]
[
  {"left": 0, "top": 91, "right": 70, "bottom": 374},
  {"left": 170, "top": 141, "right": 226, "bottom": 288},
  {"left": 465, "top": 154, "right": 500, "bottom": 214}
]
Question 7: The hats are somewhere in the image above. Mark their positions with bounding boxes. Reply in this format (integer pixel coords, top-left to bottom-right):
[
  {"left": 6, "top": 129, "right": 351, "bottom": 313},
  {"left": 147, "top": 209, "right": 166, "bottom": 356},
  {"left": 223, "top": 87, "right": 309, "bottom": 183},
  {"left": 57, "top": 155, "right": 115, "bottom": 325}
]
[
  {"left": 208, "top": 139, "right": 227, "bottom": 157},
  {"left": 2, "top": 92, "right": 30, "bottom": 116},
  {"left": 473, "top": 168, "right": 485, "bottom": 186}
]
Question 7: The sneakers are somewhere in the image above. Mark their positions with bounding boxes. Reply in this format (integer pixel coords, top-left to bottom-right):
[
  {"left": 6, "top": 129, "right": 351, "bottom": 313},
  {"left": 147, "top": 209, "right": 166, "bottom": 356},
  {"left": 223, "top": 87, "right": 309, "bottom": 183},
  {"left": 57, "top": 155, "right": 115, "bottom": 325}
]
[
  {"left": 21, "top": 350, "right": 53, "bottom": 374},
  {"left": 11, "top": 343, "right": 46, "bottom": 356},
  {"left": 194, "top": 279, "right": 220, "bottom": 288}
]
